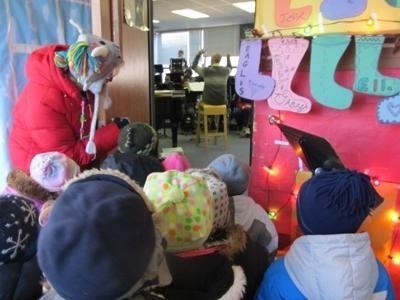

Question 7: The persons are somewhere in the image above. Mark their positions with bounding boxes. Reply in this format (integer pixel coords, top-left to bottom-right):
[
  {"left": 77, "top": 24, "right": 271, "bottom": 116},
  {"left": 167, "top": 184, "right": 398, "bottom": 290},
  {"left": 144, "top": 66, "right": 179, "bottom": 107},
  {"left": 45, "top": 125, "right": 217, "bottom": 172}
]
[
  {"left": 164, "top": 50, "right": 192, "bottom": 90},
  {"left": 254, "top": 168, "right": 395, "bottom": 300},
  {"left": 192, "top": 49, "right": 232, "bottom": 132},
  {"left": 7, "top": 18, "right": 132, "bottom": 175},
  {"left": 0, "top": 121, "right": 279, "bottom": 300}
]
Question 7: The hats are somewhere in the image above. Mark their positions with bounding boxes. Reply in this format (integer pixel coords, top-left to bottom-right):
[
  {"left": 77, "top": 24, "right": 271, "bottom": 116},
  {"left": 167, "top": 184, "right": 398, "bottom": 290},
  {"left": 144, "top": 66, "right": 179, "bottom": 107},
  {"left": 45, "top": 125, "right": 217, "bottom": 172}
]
[
  {"left": 296, "top": 169, "right": 375, "bottom": 234},
  {"left": 68, "top": 34, "right": 123, "bottom": 96},
  {"left": 29, "top": 122, "right": 247, "bottom": 300}
]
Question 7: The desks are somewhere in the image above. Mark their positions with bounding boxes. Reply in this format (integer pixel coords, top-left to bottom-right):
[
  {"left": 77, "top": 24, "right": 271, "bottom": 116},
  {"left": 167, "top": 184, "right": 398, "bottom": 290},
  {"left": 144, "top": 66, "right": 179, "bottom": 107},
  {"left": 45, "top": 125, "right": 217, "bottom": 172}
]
[{"left": 155, "top": 97, "right": 183, "bottom": 147}]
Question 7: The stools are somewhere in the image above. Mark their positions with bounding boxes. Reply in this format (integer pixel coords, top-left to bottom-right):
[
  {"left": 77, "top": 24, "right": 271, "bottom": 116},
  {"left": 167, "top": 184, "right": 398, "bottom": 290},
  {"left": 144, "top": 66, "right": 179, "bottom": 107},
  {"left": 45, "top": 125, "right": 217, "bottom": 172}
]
[{"left": 197, "top": 103, "right": 233, "bottom": 151}]
